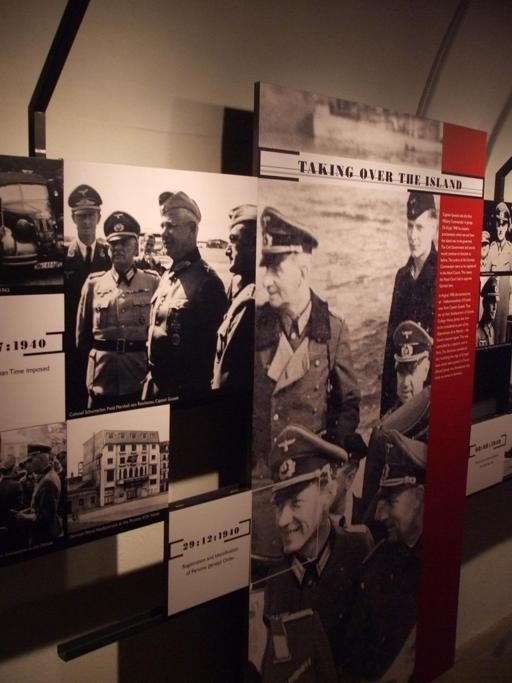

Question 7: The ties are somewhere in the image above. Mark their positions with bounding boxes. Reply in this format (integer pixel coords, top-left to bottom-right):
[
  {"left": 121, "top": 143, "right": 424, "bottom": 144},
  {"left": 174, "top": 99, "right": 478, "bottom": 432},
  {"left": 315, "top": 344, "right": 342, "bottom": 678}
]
[{"left": 84, "top": 245, "right": 92, "bottom": 267}]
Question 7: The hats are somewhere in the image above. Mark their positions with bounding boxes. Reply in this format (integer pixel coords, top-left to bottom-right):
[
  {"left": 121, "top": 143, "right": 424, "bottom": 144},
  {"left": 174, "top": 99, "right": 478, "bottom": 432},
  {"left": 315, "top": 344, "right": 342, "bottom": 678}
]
[
  {"left": 493, "top": 203, "right": 509, "bottom": 223},
  {"left": 260, "top": 208, "right": 318, "bottom": 269},
  {"left": 68, "top": 187, "right": 256, "bottom": 242},
  {"left": 266, "top": 324, "right": 433, "bottom": 507},
  {"left": 408, "top": 194, "right": 437, "bottom": 220},
  {"left": 481, "top": 230, "right": 490, "bottom": 246},
  {"left": 19, "top": 443, "right": 53, "bottom": 464}
]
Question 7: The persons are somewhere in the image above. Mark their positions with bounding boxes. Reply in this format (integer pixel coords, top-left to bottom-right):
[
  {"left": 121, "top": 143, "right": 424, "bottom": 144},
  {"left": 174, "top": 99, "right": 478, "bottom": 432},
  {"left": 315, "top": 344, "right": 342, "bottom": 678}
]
[{"left": 1, "top": 183, "right": 510, "bottom": 683}]
